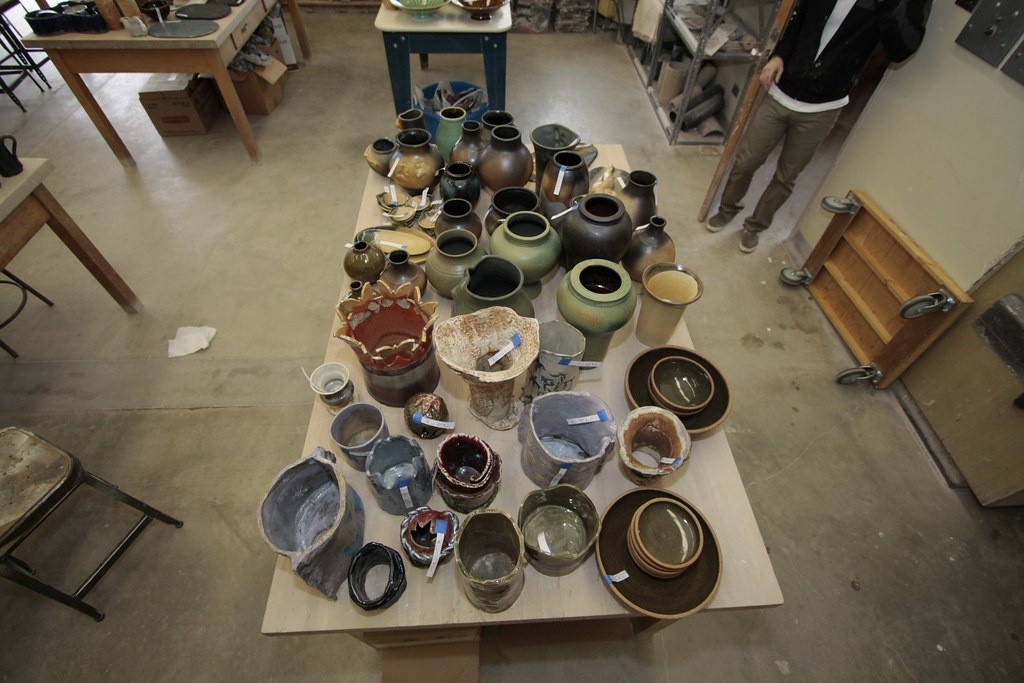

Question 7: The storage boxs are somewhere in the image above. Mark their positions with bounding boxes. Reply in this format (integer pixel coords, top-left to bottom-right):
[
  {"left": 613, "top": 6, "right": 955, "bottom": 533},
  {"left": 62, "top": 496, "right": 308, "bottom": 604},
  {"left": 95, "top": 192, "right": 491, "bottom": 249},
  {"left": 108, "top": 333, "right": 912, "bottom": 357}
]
[
  {"left": 254, "top": 37, "right": 289, "bottom": 87},
  {"left": 139, "top": 70, "right": 223, "bottom": 136},
  {"left": 266, "top": 14, "right": 298, "bottom": 70},
  {"left": 198, "top": 55, "right": 288, "bottom": 114}
]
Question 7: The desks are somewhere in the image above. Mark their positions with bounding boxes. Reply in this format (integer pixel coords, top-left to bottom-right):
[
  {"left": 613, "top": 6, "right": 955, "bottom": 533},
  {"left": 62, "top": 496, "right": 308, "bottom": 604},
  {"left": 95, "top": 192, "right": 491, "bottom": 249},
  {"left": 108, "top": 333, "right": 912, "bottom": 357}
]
[
  {"left": 0, "top": 156, "right": 141, "bottom": 315},
  {"left": 375, "top": 0, "right": 513, "bottom": 118},
  {"left": 261, "top": 144, "right": 786, "bottom": 650},
  {"left": 21, "top": 1, "right": 312, "bottom": 170}
]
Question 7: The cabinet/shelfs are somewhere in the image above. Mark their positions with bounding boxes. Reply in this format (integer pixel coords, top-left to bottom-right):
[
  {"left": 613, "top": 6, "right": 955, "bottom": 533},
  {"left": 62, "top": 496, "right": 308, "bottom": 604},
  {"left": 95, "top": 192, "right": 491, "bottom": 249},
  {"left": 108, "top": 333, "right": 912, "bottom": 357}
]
[{"left": 627, "top": 0, "right": 783, "bottom": 145}]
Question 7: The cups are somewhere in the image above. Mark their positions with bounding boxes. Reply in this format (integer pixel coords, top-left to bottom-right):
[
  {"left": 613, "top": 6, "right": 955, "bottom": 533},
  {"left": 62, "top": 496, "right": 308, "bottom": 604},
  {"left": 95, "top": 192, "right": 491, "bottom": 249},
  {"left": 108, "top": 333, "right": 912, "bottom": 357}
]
[
  {"left": 0, "top": 135, "right": 23, "bottom": 178},
  {"left": 517, "top": 483, "right": 601, "bottom": 576},
  {"left": 635, "top": 263, "right": 703, "bottom": 347},
  {"left": 330, "top": 403, "right": 389, "bottom": 471},
  {"left": 454, "top": 508, "right": 524, "bottom": 614},
  {"left": 521, "top": 391, "right": 616, "bottom": 490}
]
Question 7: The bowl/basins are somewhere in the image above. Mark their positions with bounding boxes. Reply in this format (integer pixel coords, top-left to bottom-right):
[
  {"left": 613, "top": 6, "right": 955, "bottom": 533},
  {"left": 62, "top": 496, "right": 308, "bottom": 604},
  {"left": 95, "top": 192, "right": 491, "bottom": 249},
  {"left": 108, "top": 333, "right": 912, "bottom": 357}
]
[
  {"left": 390, "top": 0, "right": 450, "bottom": 22},
  {"left": 627, "top": 498, "right": 703, "bottom": 577},
  {"left": 648, "top": 356, "right": 714, "bottom": 416},
  {"left": 450, "top": 0, "right": 509, "bottom": 20}
]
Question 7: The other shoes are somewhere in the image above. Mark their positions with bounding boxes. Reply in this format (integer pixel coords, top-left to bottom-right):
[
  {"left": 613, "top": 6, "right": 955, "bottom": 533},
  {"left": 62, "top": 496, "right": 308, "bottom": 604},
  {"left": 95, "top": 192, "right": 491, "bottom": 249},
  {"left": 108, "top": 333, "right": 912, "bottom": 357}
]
[
  {"left": 740, "top": 230, "right": 758, "bottom": 253},
  {"left": 707, "top": 211, "right": 735, "bottom": 232}
]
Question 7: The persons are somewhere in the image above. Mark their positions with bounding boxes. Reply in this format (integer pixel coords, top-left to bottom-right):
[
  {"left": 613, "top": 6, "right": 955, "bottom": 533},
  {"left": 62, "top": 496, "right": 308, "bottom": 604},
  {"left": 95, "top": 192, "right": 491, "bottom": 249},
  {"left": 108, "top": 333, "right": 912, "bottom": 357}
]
[{"left": 707, "top": 0, "right": 932, "bottom": 252}]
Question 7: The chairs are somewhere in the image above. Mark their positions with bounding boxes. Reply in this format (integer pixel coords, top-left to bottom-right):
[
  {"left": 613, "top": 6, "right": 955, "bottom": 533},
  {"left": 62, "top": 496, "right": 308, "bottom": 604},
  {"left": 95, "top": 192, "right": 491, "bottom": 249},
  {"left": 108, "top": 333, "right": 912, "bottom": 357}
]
[{"left": 0, "top": 427, "right": 183, "bottom": 622}]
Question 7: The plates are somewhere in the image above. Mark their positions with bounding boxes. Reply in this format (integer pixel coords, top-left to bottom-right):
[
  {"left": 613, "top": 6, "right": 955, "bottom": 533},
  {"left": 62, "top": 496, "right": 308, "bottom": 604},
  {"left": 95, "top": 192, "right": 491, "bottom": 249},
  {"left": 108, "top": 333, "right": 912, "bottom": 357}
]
[{"left": 353, "top": 192, "right": 443, "bottom": 264}]
